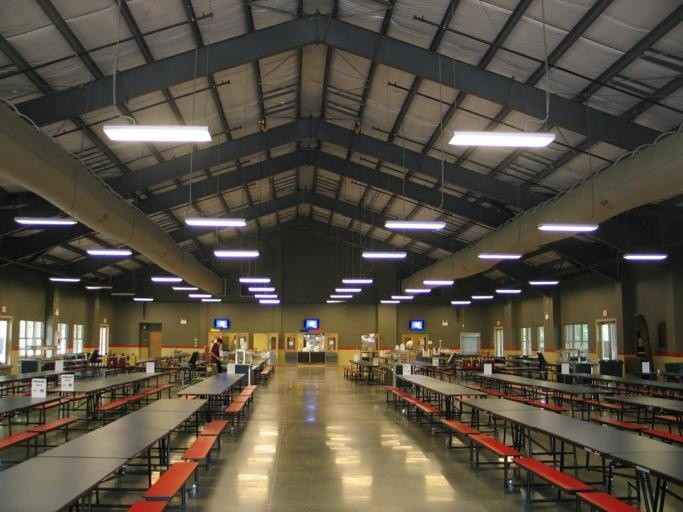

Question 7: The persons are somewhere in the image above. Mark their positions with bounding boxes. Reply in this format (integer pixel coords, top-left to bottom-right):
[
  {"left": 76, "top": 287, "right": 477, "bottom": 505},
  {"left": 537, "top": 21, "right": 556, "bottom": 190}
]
[
  {"left": 89, "top": 348, "right": 102, "bottom": 362},
  {"left": 210, "top": 338, "right": 222, "bottom": 372},
  {"left": 406, "top": 337, "right": 413, "bottom": 350}
]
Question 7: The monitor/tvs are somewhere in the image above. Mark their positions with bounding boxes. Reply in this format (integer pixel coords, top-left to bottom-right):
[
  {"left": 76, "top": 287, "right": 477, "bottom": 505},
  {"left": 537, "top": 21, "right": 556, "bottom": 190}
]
[
  {"left": 304, "top": 319, "right": 319, "bottom": 329},
  {"left": 214, "top": 319, "right": 230, "bottom": 329},
  {"left": 409, "top": 320, "right": 424, "bottom": 330}
]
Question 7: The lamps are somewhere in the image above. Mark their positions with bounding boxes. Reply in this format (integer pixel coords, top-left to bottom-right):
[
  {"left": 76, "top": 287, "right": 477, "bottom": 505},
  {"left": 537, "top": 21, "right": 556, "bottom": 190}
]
[
  {"left": 103, "top": 0, "right": 215, "bottom": 141},
  {"left": 447, "top": 0, "right": 556, "bottom": 147}
]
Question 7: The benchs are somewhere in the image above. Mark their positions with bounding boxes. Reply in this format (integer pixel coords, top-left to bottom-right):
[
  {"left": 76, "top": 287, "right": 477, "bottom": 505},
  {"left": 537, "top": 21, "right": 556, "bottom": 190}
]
[
  {"left": 0, "top": 354, "right": 274, "bottom": 512},
  {"left": 342, "top": 356, "right": 683, "bottom": 511}
]
